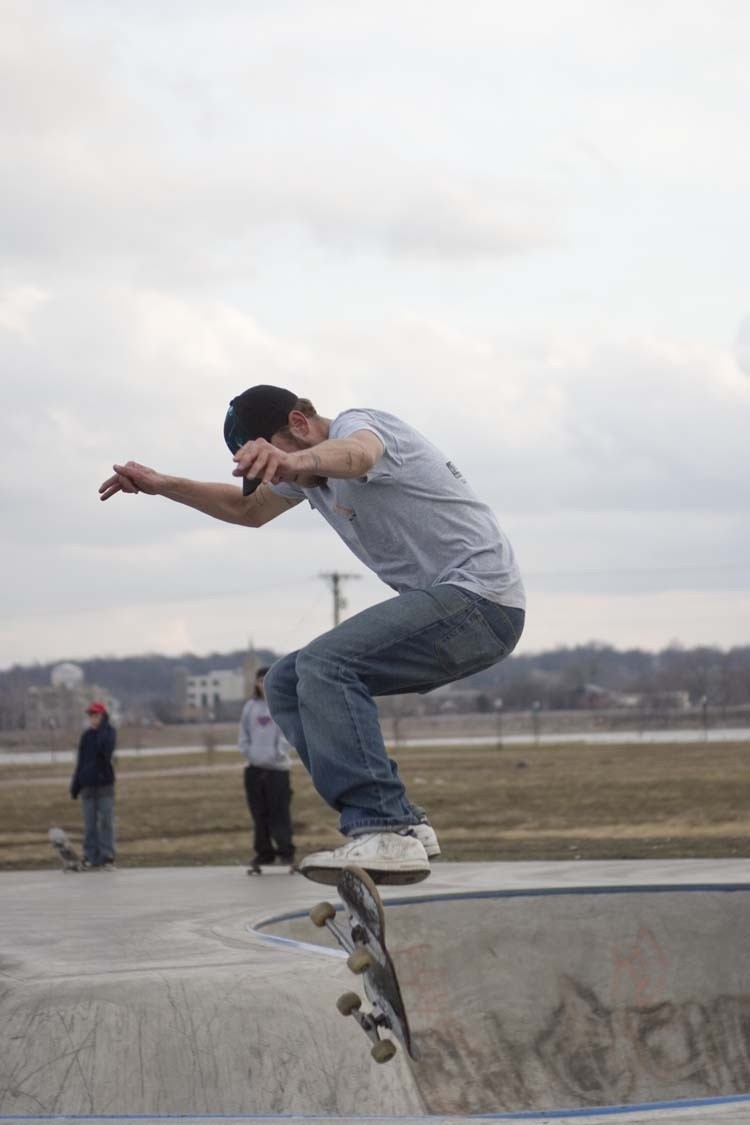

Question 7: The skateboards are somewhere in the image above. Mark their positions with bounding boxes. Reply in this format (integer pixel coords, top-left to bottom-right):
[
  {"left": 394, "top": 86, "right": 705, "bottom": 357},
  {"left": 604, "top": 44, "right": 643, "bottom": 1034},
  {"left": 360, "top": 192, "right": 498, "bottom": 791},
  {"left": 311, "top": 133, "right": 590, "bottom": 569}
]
[
  {"left": 309, "top": 864, "right": 422, "bottom": 1064},
  {"left": 48, "top": 827, "right": 82, "bottom": 873},
  {"left": 238, "top": 862, "right": 297, "bottom": 876}
]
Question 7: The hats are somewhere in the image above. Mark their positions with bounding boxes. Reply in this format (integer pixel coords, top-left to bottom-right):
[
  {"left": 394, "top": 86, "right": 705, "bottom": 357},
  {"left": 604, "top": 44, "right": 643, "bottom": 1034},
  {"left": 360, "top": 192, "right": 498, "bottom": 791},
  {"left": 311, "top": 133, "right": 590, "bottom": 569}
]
[
  {"left": 223, "top": 385, "right": 297, "bottom": 497},
  {"left": 86, "top": 704, "right": 105, "bottom": 712}
]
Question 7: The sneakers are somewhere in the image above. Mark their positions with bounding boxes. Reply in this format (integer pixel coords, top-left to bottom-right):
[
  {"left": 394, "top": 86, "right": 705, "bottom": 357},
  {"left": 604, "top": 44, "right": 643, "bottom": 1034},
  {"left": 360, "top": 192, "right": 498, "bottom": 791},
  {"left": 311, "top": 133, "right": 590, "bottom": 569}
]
[
  {"left": 410, "top": 823, "right": 441, "bottom": 857},
  {"left": 299, "top": 829, "right": 431, "bottom": 887}
]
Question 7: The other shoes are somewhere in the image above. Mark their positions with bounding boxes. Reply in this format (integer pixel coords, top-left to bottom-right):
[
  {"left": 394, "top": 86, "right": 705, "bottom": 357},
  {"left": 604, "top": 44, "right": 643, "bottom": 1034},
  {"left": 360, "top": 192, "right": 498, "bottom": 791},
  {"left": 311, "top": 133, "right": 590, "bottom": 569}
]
[
  {"left": 104, "top": 861, "right": 118, "bottom": 872},
  {"left": 251, "top": 854, "right": 274, "bottom": 865},
  {"left": 280, "top": 853, "right": 295, "bottom": 865},
  {"left": 82, "top": 858, "right": 100, "bottom": 871}
]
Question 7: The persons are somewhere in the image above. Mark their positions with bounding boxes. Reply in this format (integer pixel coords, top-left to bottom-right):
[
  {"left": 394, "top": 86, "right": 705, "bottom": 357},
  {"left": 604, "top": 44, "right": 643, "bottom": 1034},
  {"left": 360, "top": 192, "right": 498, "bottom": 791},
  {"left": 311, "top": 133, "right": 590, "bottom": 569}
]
[
  {"left": 237, "top": 667, "right": 298, "bottom": 875},
  {"left": 68, "top": 702, "right": 117, "bottom": 874},
  {"left": 97, "top": 385, "right": 525, "bottom": 886}
]
